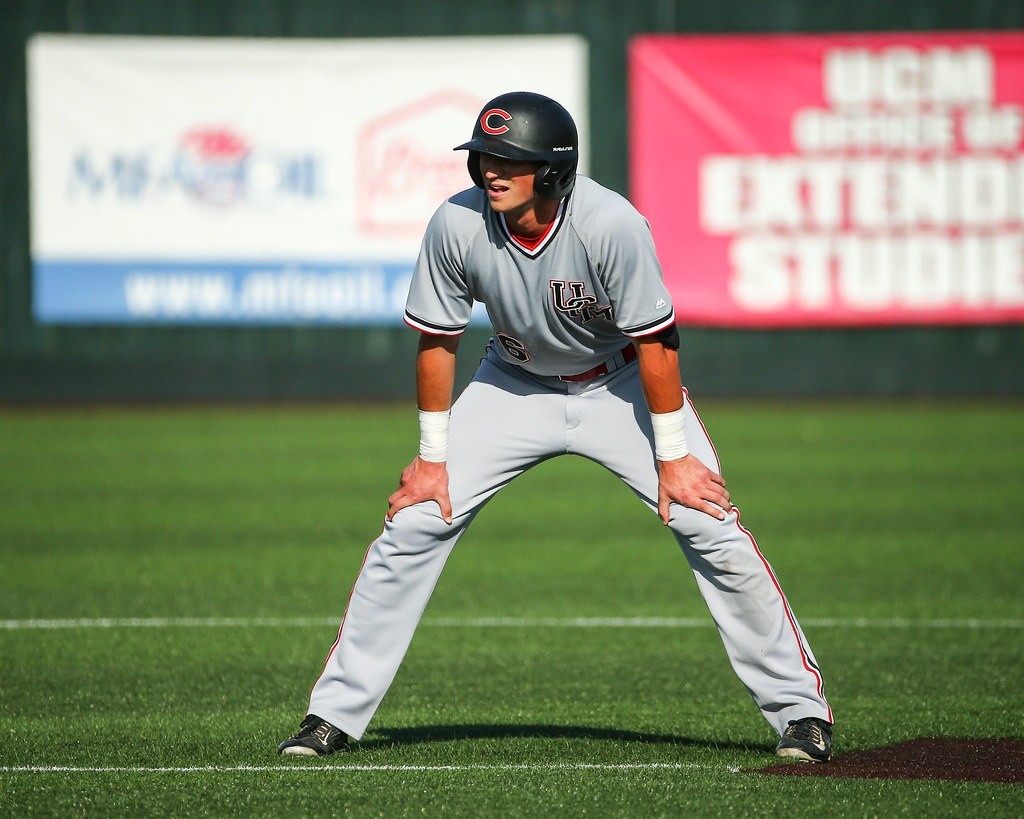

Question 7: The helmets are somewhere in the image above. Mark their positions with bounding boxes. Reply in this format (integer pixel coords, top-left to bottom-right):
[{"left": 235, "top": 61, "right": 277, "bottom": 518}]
[{"left": 453, "top": 92, "right": 579, "bottom": 200}]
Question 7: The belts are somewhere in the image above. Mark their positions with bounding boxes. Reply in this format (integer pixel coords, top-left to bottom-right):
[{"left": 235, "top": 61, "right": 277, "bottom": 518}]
[{"left": 554, "top": 342, "right": 638, "bottom": 382}]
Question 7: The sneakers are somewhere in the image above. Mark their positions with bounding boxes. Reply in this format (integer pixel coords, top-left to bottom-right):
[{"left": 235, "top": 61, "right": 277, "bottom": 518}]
[
  {"left": 775, "top": 714, "right": 833, "bottom": 763},
  {"left": 278, "top": 714, "right": 350, "bottom": 756}
]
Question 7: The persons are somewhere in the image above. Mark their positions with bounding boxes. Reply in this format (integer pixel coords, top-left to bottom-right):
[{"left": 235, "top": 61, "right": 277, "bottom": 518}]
[{"left": 279, "top": 91, "right": 834, "bottom": 762}]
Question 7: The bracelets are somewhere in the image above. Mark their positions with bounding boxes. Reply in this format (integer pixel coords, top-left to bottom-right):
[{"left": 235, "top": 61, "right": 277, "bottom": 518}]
[
  {"left": 415, "top": 406, "right": 452, "bottom": 464},
  {"left": 648, "top": 402, "right": 689, "bottom": 463}
]
